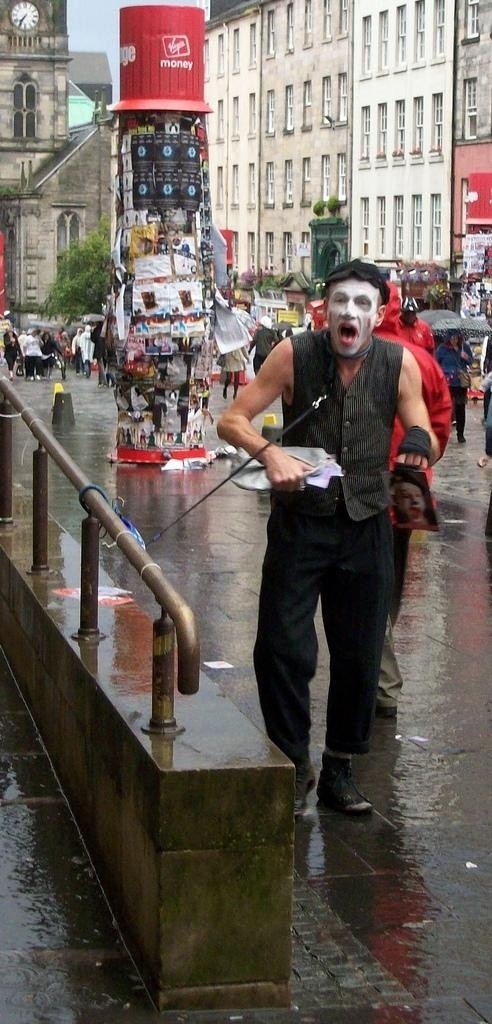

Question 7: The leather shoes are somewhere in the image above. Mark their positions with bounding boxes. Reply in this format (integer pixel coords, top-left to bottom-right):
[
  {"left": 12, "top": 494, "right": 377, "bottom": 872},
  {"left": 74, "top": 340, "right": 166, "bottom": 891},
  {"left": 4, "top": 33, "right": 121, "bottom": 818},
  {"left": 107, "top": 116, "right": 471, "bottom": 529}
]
[{"left": 222, "top": 388, "right": 239, "bottom": 401}]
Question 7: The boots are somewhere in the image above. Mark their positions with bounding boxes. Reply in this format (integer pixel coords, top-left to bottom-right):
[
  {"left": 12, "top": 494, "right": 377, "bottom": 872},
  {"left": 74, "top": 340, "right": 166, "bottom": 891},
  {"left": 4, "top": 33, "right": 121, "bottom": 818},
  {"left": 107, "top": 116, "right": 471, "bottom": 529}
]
[
  {"left": 293, "top": 755, "right": 317, "bottom": 817},
  {"left": 315, "top": 751, "right": 373, "bottom": 816}
]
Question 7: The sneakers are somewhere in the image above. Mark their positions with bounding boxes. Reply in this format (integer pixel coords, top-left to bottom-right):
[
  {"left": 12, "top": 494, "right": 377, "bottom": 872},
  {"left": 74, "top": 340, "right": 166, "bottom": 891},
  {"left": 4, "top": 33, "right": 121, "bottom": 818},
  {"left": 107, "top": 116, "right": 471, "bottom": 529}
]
[
  {"left": 29, "top": 375, "right": 41, "bottom": 382},
  {"left": 456, "top": 434, "right": 467, "bottom": 445},
  {"left": 373, "top": 703, "right": 398, "bottom": 719}
]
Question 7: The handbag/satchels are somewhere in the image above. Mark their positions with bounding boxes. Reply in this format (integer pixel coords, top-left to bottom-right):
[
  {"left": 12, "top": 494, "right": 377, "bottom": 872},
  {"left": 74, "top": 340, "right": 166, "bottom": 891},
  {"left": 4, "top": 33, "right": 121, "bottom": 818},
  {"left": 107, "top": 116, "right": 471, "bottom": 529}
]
[{"left": 459, "top": 367, "right": 472, "bottom": 389}]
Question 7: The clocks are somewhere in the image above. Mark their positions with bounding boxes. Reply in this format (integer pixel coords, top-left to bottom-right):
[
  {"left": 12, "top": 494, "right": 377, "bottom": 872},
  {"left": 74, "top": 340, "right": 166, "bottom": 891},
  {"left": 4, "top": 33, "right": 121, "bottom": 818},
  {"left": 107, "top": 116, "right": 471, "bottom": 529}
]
[{"left": 8, "top": 0, "right": 40, "bottom": 30}]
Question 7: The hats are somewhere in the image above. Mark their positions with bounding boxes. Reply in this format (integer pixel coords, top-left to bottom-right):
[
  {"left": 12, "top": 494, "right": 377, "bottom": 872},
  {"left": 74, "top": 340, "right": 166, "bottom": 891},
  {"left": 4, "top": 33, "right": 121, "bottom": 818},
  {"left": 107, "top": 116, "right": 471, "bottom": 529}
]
[
  {"left": 400, "top": 295, "right": 421, "bottom": 313},
  {"left": 323, "top": 258, "right": 391, "bottom": 306}
]
